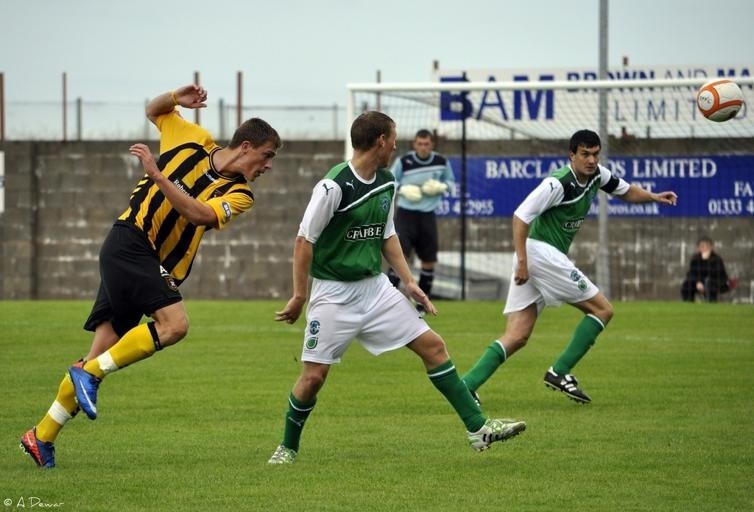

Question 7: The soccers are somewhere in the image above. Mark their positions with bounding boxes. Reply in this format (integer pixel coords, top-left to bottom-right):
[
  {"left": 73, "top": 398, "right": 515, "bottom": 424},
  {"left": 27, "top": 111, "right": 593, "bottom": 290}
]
[{"left": 697, "top": 80, "right": 743, "bottom": 122}]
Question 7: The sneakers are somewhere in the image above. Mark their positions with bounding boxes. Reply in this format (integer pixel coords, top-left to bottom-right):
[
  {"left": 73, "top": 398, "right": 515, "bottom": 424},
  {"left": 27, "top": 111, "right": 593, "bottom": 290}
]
[
  {"left": 68, "top": 359, "right": 99, "bottom": 420},
  {"left": 466, "top": 418, "right": 526, "bottom": 452},
  {"left": 20, "top": 427, "right": 56, "bottom": 468},
  {"left": 543, "top": 365, "right": 592, "bottom": 404},
  {"left": 416, "top": 304, "right": 427, "bottom": 318},
  {"left": 268, "top": 444, "right": 297, "bottom": 465}
]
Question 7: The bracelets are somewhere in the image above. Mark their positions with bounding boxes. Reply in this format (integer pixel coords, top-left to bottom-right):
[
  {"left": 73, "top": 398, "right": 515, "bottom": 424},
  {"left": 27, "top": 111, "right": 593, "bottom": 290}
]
[{"left": 171, "top": 91, "right": 178, "bottom": 105}]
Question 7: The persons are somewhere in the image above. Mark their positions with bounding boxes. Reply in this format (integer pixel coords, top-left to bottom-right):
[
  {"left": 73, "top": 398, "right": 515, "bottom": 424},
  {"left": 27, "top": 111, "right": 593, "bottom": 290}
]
[
  {"left": 681, "top": 236, "right": 729, "bottom": 302},
  {"left": 385, "top": 130, "right": 455, "bottom": 317},
  {"left": 460, "top": 128, "right": 678, "bottom": 407},
  {"left": 20, "top": 85, "right": 283, "bottom": 470},
  {"left": 267, "top": 111, "right": 526, "bottom": 467}
]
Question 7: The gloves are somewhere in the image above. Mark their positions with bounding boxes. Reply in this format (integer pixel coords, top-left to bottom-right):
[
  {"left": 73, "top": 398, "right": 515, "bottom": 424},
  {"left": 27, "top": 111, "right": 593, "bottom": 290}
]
[
  {"left": 400, "top": 185, "right": 422, "bottom": 202},
  {"left": 423, "top": 179, "right": 448, "bottom": 196}
]
